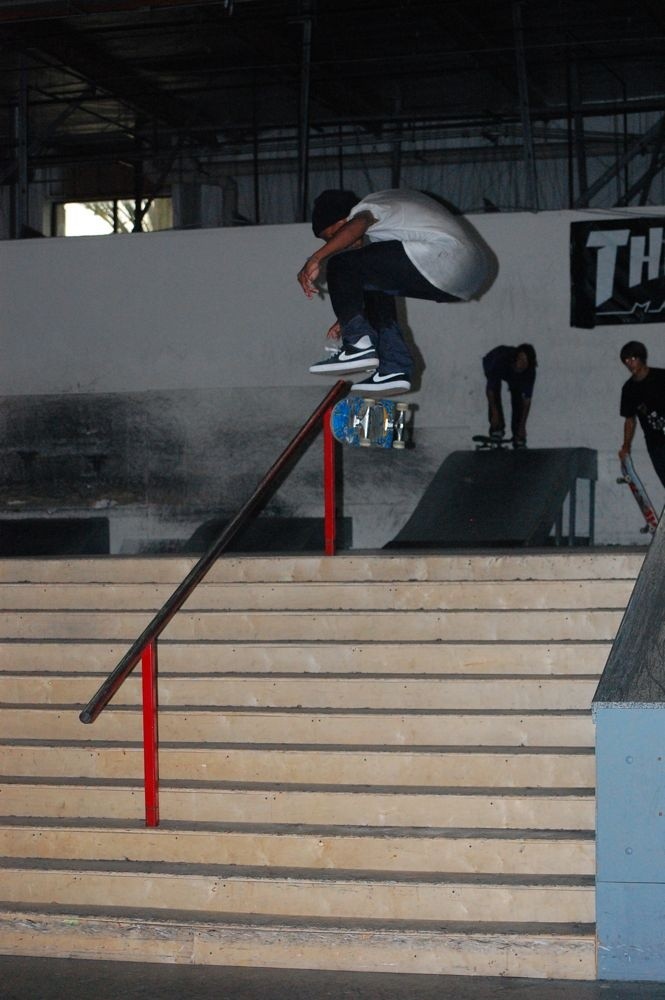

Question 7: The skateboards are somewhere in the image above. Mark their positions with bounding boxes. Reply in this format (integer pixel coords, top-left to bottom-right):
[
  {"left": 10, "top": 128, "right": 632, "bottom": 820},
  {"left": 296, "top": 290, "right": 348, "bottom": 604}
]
[
  {"left": 473, "top": 436, "right": 514, "bottom": 450},
  {"left": 616, "top": 453, "right": 659, "bottom": 534},
  {"left": 329, "top": 396, "right": 407, "bottom": 449}
]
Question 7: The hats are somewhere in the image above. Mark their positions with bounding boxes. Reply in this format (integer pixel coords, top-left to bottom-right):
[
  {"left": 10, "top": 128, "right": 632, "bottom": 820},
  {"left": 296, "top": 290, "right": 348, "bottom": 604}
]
[
  {"left": 620, "top": 342, "right": 648, "bottom": 363},
  {"left": 311, "top": 189, "right": 361, "bottom": 237}
]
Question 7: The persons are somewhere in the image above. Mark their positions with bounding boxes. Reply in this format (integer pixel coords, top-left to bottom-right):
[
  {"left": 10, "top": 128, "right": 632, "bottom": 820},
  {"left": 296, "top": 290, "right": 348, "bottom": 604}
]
[
  {"left": 617, "top": 340, "right": 665, "bottom": 487},
  {"left": 482, "top": 343, "right": 538, "bottom": 449},
  {"left": 296, "top": 187, "right": 487, "bottom": 396}
]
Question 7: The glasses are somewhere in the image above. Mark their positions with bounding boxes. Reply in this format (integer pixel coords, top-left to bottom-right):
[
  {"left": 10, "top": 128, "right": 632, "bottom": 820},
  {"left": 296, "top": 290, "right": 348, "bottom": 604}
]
[{"left": 625, "top": 357, "right": 638, "bottom": 363}]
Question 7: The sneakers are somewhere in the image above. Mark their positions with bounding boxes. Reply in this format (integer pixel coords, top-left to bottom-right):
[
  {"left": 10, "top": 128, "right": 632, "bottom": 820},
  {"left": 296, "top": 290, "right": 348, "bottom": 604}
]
[
  {"left": 309, "top": 346, "right": 380, "bottom": 375},
  {"left": 351, "top": 372, "right": 410, "bottom": 397}
]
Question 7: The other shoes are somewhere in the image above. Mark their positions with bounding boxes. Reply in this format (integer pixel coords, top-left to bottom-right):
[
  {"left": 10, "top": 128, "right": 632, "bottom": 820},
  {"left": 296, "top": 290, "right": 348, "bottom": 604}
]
[
  {"left": 489, "top": 428, "right": 505, "bottom": 437},
  {"left": 512, "top": 439, "right": 525, "bottom": 448}
]
